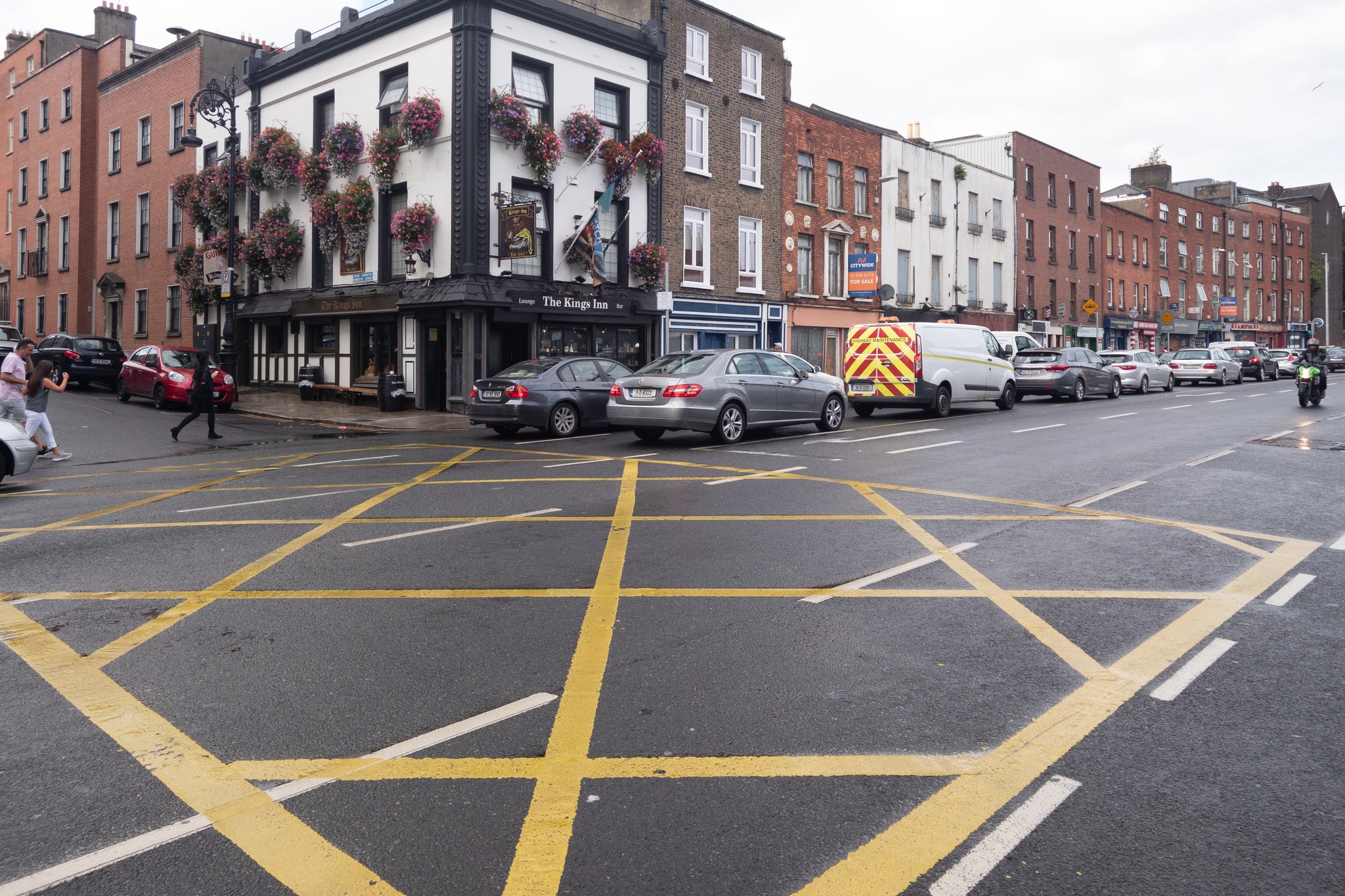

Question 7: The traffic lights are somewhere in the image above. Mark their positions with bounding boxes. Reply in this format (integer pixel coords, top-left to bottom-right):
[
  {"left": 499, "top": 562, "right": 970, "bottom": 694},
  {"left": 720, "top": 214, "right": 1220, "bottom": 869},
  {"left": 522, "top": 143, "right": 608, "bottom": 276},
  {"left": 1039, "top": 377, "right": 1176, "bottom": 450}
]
[
  {"left": 1307, "top": 324, "right": 1312, "bottom": 334},
  {"left": 1313, "top": 323, "right": 1317, "bottom": 334}
]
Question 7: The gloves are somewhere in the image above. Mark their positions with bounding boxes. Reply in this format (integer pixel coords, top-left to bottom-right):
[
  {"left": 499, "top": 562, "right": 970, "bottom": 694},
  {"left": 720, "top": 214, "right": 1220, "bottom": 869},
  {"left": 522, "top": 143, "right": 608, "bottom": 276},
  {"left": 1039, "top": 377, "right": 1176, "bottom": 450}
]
[
  {"left": 1292, "top": 361, "right": 1299, "bottom": 365},
  {"left": 1320, "top": 362, "right": 1325, "bottom": 365}
]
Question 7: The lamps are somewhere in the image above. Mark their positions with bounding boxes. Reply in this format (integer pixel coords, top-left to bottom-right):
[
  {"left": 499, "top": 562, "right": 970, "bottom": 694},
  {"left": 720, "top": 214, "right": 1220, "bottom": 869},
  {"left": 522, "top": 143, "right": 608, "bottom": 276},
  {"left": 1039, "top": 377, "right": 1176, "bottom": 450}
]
[{"left": 404, "top": 248, "right": 431, "bottom": 276}]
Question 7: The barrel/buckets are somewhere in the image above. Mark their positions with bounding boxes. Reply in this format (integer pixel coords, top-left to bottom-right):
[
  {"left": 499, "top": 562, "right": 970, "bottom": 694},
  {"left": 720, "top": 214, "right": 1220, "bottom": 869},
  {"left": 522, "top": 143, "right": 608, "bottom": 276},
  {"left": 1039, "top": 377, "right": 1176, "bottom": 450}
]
[
  {"left": 298, "top": 366, "right": 324, "bottom": 401},
  {"left": 377, "top": 373, "right": 406, "bottom": 412}
]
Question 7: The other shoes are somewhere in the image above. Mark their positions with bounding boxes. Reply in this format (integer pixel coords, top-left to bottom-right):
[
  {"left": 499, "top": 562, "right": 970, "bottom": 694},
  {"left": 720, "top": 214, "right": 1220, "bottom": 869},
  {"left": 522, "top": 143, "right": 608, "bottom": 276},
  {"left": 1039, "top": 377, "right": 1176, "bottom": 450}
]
[
  {"left": 170, "top": 427, "right": 181, "bottom": 441},
  {"left": 208, "top": 430, "right": 223, "bottom": 439}
]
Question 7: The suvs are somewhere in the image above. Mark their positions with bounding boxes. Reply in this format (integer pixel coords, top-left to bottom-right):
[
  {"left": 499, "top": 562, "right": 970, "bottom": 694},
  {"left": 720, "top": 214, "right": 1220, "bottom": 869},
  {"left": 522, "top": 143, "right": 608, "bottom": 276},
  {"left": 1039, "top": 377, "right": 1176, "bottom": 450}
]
[
  {"left": 22, "top": 333, "right": 127, "bottom": 392},
  {"left": 0, "top": 320, "right": 23, "bottom": 358}
]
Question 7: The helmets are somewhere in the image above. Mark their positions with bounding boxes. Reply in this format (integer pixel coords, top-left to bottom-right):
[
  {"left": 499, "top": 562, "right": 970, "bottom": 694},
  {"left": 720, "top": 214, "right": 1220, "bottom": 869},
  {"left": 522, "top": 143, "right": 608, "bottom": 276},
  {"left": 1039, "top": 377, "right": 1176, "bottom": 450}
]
[{"left": 1306, "top": 338, "right": 1320, "bottom": 352}]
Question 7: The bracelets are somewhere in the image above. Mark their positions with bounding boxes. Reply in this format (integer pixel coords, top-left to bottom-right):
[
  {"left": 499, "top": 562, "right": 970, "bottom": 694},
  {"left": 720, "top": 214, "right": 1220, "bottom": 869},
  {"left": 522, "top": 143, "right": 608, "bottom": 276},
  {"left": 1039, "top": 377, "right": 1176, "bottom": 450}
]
[{"left": 63, "top": 377, "right": 68, "bottom": 380}]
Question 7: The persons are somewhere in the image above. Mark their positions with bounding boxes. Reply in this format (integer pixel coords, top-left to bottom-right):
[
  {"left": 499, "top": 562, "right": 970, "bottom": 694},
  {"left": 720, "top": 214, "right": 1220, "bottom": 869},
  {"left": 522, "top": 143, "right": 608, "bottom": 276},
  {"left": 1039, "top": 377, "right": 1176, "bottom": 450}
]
[
  {"left": 1131, "top": 347, "right": 1136, "bottom": 350},
  {"left": 0, "top": 339, "right": 59, "bottom": 457},
  {"left": 1163, "top": 347, "right": 1168, "bottom": 352},
  {"left": 1293, "top": 338, "right": 1330, "bottom": 399},
  {"left": 170, "top": 349, "right": 223, "bottom": 441},
  {"left": 1107, "top": 347, "right": 1113, "bottom": 350},
  {"left": 1264, "top": 343, "right": 1270, "bottom": 350},
  {"left": 22, "top": 360, "right": 73, "bottom": 461}
]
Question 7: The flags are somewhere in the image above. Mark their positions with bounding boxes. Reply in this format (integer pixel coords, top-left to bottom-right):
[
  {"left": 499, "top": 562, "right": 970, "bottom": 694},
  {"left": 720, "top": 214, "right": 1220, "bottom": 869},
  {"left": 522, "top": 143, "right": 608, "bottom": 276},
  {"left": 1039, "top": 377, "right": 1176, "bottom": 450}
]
[
  {"left": 597, "top": 155, "right": 640, "bottom": 213},
  {"left": 574, "top": 208, "right": 608, "bottom": 289}
]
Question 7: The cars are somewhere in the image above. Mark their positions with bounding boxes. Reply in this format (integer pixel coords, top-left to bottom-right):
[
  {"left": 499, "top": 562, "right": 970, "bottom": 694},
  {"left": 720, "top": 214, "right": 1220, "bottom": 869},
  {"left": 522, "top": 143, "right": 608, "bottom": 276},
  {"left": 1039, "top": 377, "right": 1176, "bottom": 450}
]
[
  {"left": 1296, "top": 341, "right": 1345, "bottom": 382},
  {"left": 729, "top": 351, "right": 844, "bottom": 390},
  {"left": 1155, "top": 351, "right": 1190, "bottom": 364},
  {"left": 605, "top": 348, "right": 849, "bottom": 444},
  {"left": 116, "top": 344, "right": 236, "bottom": 411},
  {"left": 1223, "top": 347, "right": 1279, "bottom": 382},
  {"left": 467, "top": 355, "right": 635, "bottom": 439},
  {"left": 1169, "top": 347, "right": 1243, "bottom": 386},
  {"left": 1266, "top": 348, "right": 1306, "bottom": 379},
  {"left": 1093, "top": 349, "right": 1174, "bottom": 395},
  {"left": 1010, "top": 347, "right": 1122, "bottom": 403},
  {"left": 0, "top": 417, "right": 39, "bottom": 484}
]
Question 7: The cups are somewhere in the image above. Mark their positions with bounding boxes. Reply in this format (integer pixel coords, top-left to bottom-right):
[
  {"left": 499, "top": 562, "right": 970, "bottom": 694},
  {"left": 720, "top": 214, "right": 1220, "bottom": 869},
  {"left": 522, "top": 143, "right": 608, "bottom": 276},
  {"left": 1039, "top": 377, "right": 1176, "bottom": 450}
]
[
  {"left": 629, "top": 347, "right": 635, "bottom": 352},
  {"left": 574, "top": 343, "right": 578, "bottom": 352},
  {"left": 627, "top": 360, "right": 630, "bottom": 365},
  {"left": 564, "top": 346, "right": 569, "bottom": 352},
  {"left": 307, "top": 364, "right": 312, "bottom": 367},
  {"left": 389, "top": 370, "right": 394, "bottom": 374}
]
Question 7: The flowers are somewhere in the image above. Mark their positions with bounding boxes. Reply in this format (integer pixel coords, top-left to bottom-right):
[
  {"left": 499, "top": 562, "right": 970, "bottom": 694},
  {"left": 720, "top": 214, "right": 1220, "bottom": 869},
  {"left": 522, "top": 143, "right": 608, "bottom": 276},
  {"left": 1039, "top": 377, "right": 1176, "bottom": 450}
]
[
  {"left": 168, "top": 86, "right": 439, "bottom": 317},
  {"left": 487, "top": 85, "right": 668, "bottom": 201},
  {"left": 626, "top": 241, "right": 671, "bottom": 297}
]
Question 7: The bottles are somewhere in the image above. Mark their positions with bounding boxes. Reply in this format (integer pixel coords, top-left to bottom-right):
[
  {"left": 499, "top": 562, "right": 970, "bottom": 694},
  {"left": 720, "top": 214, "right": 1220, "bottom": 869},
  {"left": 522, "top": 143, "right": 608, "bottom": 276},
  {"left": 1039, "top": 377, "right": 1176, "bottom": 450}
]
[
  {"left": 539, "top": 341, "right": 545, "bottom": 352},
  {"left": 569, "top": 344, "right": 573, "bottom": 352},
  {"left": 545, "top": 344, "right": 549, "bottom": 352},
  {"left": 596, "top": 342, "right": 598, "bottom": 352},
  {"left": 635, "top": 360, "right": 638, "bottom": 365},
  {"left": 606, "top": 344, "right": 609, "bottom": 352}
]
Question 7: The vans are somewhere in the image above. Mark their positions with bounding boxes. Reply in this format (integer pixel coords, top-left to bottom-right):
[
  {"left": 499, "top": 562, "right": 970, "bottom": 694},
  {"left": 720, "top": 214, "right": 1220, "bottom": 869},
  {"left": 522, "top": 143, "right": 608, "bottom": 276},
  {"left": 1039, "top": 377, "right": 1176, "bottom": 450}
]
[
  {"left": 843, "top": 316, "right": 1016, "bottom": 419},
  {"left": 983, "top": 331, "right": 1056, "bottom": 362},
  {"left": 1208, "top": 341, "right": 1260, "bottom": 354}
]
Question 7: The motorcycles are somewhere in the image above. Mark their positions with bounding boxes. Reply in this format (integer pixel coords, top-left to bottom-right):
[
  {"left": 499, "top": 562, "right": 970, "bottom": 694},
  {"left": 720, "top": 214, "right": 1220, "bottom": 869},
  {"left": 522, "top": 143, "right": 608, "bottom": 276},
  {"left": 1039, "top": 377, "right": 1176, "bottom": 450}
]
[{"left": 1291, "top": 362, "right": 1326, "bottom": 407}]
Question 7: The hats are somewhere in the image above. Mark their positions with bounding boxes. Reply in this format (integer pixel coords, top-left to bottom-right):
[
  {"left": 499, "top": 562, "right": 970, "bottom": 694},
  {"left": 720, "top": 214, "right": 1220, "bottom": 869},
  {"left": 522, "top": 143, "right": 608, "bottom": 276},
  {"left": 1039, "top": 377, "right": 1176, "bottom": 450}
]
[
  {"left": 774, "top": 342, "right": 782, "bottom": 348},
  {"left": 1164, "top": 346, "right": 1168, "bottom": 348},
  {"left": 1260, "top": 345, "right": 1263, "bottom": 347},
  {"left": 1161, "top": 350, "right": 1165, "bottom": 352}
]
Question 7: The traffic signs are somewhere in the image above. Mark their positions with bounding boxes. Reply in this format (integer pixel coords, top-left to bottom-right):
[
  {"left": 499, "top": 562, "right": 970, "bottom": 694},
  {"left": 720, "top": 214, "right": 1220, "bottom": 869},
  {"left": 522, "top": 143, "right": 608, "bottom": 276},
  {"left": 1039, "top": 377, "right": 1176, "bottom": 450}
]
[{"left": 1058, "top": 302, "right": 1065, "bottom": 316}]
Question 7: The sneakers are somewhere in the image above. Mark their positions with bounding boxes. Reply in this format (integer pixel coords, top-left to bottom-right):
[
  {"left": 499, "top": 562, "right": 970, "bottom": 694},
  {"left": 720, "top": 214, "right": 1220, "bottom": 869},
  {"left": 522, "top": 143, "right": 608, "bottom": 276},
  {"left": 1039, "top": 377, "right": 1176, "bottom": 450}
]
[
  {"left": 52, "top": 451, "right": 72, "bottom": 461},
  {"left": 1319, "top": 390, "right": 1325, "bottom": 399},
  {"left": 37, "top": 444, "right": 59, "bottom": 457}
]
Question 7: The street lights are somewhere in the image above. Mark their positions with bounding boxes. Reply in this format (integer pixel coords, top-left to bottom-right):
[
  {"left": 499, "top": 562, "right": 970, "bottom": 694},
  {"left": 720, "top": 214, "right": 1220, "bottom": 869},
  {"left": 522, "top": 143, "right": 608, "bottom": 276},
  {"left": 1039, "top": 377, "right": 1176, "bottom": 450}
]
[{"left": 179, "top": 65, "right": 240, "bottom": 403}]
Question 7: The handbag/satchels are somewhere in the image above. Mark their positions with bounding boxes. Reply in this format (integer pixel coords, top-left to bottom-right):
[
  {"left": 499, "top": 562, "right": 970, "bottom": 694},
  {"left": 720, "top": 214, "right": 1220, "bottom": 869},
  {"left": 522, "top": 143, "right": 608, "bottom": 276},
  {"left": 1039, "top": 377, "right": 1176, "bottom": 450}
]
[{"left": 188, "top": 391, "right": 193, "bottom": 407}]
